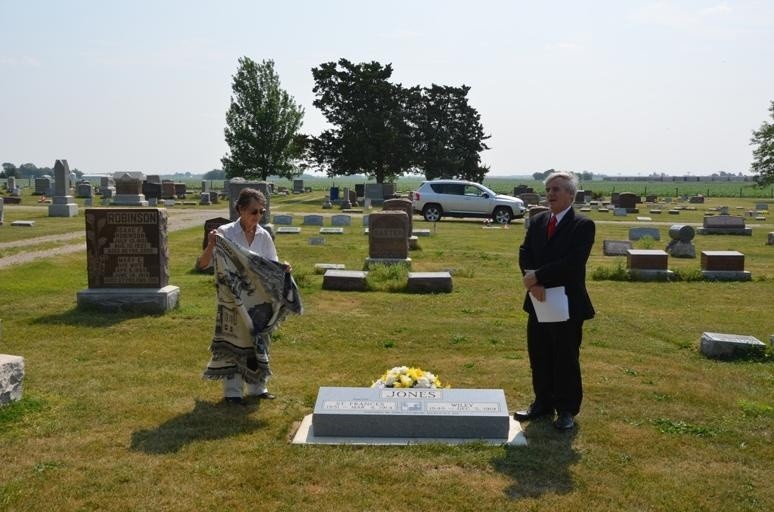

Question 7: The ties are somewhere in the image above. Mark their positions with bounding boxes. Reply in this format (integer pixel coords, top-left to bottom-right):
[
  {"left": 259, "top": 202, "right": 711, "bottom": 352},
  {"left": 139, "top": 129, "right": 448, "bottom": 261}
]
[{"left": 548, "top": 215, "right": 558, "bottom": 238}]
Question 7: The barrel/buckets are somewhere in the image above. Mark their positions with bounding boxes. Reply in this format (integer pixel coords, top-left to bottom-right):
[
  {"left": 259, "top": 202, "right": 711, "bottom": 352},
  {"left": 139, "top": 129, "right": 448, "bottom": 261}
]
[{"left": 670, "top": 224, "right": 695, "bottom": 241}]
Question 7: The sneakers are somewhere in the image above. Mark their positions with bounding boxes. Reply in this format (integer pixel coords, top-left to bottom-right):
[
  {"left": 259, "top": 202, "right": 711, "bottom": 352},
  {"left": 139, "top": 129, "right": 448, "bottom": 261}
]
[
  {"left": 224, "top": 397, "right": 247, "bottom": 406},
  {"left": 257, "top": 392, "right": 276, "bottom": 400}
]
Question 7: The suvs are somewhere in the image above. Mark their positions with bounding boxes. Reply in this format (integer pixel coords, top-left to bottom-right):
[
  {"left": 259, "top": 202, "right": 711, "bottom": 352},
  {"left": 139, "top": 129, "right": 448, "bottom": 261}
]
[{"left": 412, "top": 180, "right": 526, "bottom": 225}]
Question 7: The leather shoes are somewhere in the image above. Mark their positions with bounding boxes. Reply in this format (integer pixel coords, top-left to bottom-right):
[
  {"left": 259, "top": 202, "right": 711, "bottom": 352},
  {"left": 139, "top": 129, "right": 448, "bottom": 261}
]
[
  {"left": 514, "top": 403, "right": 554, "bottom": 421},
  {"left": 554, "top": 411, "right": 574, "bottom": 430}
]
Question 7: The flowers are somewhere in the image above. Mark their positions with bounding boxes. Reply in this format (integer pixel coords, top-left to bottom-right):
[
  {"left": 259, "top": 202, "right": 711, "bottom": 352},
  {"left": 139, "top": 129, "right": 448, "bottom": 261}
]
[{"left": 371, "top": 365, "right": 451, "bottom": 390}]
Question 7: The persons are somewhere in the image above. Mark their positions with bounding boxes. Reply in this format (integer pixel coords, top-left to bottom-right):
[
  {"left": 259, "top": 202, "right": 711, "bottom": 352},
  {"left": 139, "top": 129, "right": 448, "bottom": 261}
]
[
  {"left": 513, "top": 171, "right": 596, "bottom": 431},
  {"left": 197, "top": 187, "right": 293, "bottom": 404}
]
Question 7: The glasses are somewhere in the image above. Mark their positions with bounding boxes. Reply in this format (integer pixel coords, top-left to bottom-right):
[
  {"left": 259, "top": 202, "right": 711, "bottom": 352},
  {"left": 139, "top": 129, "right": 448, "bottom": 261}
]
[{"left": 247, "top": 206, "right": 266, "bottom": 215}]
